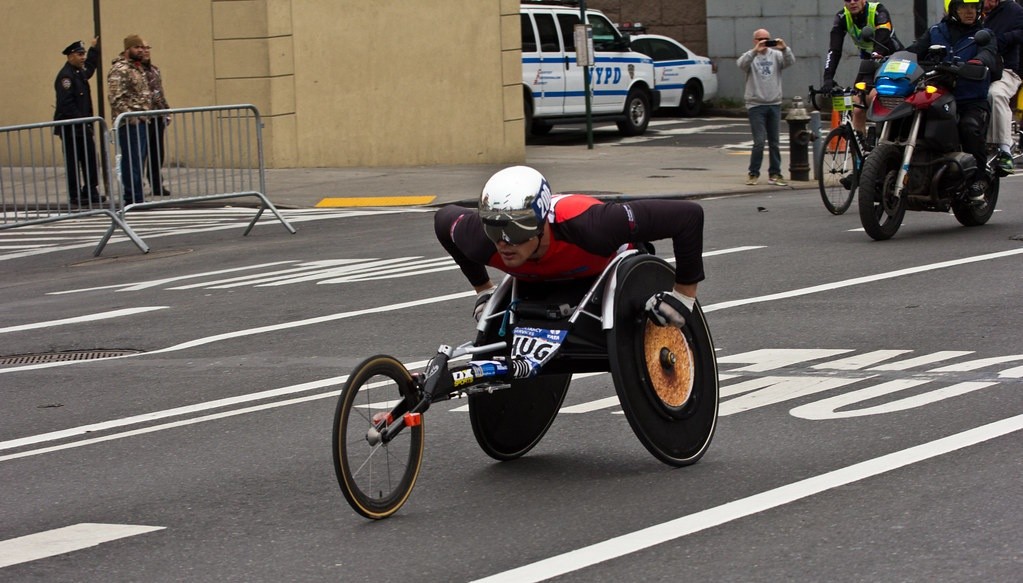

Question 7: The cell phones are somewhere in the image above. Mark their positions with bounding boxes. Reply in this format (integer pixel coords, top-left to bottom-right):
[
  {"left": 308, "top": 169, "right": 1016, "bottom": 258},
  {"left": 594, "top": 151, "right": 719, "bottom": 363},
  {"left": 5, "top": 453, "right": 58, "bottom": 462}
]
[{"left": 765, "top": 41, "right": 778, "bottom": 46}]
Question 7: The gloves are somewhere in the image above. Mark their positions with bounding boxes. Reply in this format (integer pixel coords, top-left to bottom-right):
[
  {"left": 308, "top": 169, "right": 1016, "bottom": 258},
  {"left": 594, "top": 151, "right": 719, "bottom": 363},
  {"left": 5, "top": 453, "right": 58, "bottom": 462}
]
[
  {"left": 963, "top": 63, "right": 984, "bottom": 77},
  {"left": 644, "top": 291, "right": 695, "bottom": 328},
  {"left": 472, "top": 286, "right": 497, "bottom": 321}
]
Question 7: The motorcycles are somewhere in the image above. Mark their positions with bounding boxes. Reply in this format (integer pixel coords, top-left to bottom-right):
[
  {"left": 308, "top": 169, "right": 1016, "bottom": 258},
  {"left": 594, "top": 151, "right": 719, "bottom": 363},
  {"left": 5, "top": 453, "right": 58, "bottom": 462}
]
[{"left": 855, "top": 24, "right": 1023, "bottom": 242}]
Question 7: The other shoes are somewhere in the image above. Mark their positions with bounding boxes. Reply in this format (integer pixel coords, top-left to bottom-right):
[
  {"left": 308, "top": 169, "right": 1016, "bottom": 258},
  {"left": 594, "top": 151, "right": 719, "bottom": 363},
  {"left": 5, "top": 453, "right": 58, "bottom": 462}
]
[
  {"left": 996, "top": 151, "right": 1014, "bottom": 175},
  {"left": 840, "top": 175, "right": 858, "bottom": 187},
  {"left": 70, "top": 198, "right": 88, "bottom": 205},
  {"left": 968, "top": 174, "right": 991, "bottom": 197},
  {"left": 768, "top": 176, "right": 788, "bottom": 186},
  {"left": 151, "top": 186, "right": 171, "bottom": 196},
  {"left": 745, "top": 175, "right": 758, "bottom": 184},
  {"left": 124, "top": 199, "right": 153, "bottom": 209},
  {"left": 82, "top": 187, "right": 106, "bottom": 202}
]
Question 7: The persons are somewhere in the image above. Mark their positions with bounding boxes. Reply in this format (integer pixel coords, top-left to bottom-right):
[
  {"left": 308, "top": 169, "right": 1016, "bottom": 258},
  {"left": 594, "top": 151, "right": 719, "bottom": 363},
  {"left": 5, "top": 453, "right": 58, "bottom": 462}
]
[
  {"left": 736, "top": 28, "right": 795, "bottom": 186},
  {"left": 107, "top": 35, "right": 170, "bottom": 208},
  {"left": 822, "top": 0, "right": 905, "bottom": 189},
  {"left": 432, "top": 165, "right": 707, "bottom": 329},
  {"left": 53, "top": 35, "right": 107, "bottom": 205},
  {"left": 902, "top": 0, "right": 1023, "bottom": 175}
]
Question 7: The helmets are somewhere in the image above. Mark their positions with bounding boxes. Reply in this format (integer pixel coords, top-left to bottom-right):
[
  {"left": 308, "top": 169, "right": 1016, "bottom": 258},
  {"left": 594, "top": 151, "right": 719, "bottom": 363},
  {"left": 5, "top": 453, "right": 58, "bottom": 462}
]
[
  {"left": 944, "top": 0, "right": 983, "bottom": 17},
  {"left": 479, "top": 166, "right": 551, "bottom": 221}
]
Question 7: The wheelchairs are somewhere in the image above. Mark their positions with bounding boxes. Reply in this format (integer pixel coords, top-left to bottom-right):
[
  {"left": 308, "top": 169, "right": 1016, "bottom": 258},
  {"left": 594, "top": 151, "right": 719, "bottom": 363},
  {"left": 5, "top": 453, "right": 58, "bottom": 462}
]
[{"left": 332, "top": 244, "right": 702, "bottom": 520}]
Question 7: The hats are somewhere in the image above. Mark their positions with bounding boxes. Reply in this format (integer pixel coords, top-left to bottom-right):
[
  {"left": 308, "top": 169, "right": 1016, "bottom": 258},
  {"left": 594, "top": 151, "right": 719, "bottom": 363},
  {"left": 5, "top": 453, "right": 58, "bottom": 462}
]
[
  {"left": 62, "top": 40, "right": 88, "bottom": 55},
  {"left": 123, "top": 34, "right": 144, "bottom": 50}
]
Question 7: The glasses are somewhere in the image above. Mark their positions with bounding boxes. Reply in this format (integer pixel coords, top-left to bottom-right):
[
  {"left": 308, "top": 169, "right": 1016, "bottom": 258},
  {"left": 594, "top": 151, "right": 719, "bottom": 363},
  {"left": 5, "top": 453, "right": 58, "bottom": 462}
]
[
  {"left": 484, "top": 217, "right": 540, "bottom": 246},
  {"left": 755, "top": 38, "right": 769, "bottom": 41},
  {"left": 845, "top": 0, "right": 858, "bottom": 2}
]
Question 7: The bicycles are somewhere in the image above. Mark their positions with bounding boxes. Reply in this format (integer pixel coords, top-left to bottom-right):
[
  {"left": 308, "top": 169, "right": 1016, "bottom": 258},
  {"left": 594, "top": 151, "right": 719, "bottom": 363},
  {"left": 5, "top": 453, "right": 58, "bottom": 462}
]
[{"left": 806, "top": 84, "right": 882, "bottom": 216}]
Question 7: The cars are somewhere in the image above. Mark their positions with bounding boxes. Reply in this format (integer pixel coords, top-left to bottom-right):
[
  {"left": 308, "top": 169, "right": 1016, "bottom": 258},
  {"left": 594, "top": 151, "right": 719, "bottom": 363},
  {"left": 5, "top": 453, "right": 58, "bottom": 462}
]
[{"left": 613, "top": 22, "right": 718, "bottom": 119}]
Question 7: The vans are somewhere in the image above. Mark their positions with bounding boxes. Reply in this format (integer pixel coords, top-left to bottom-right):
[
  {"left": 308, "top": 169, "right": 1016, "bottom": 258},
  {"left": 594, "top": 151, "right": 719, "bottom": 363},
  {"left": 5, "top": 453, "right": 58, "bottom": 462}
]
[{"left": 520, "top": 0, "right": 661, "bottom": 146}]
